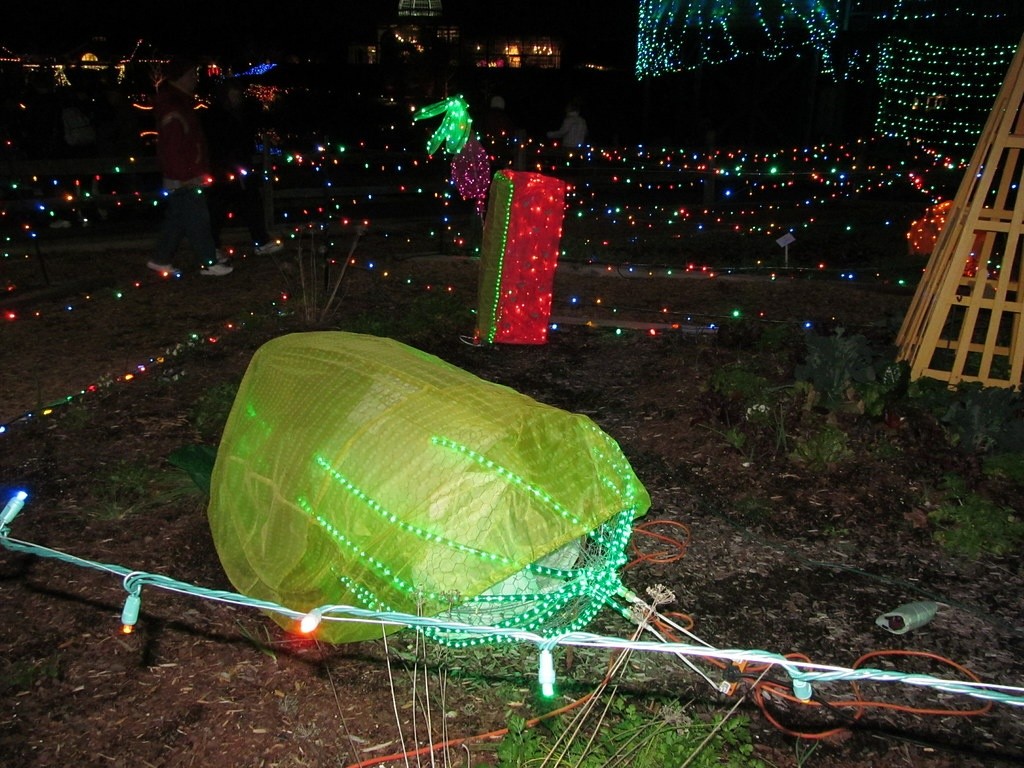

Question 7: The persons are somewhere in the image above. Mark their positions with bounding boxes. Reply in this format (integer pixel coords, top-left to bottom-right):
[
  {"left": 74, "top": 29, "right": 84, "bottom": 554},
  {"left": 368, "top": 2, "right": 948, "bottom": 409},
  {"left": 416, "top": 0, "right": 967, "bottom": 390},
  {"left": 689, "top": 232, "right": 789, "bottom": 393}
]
[
  {"left": 543, "top": 102, "right": 588, "bottom": 148},
  {"left": 702, "top": 128, "right": 720, "bottom": 154},
  {"left": 146, "top": 56, "right": 234, "bottom": 277},
  {"left": 204, "top": 77, "right": 285, "bottom": 264},
  {"left": 476, "top": 96, "right": 515, "bottom": 170}
]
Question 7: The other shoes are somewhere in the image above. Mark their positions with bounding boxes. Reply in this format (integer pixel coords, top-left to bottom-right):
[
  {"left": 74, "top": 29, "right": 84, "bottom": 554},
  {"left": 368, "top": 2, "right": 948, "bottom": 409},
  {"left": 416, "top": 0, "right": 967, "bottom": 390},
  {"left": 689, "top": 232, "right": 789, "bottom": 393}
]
[
  {"left": 147, "top": 262, "right": 179, "bottom": 274},
  {"left": 254, "top": 241, "right": 284, "bottom": 256},
  {"left": 216, "top": 249, "right": 228, "bottom": 263},
  {"left": 200, "top": 263, "right": 233, "bottom": 276}
]
